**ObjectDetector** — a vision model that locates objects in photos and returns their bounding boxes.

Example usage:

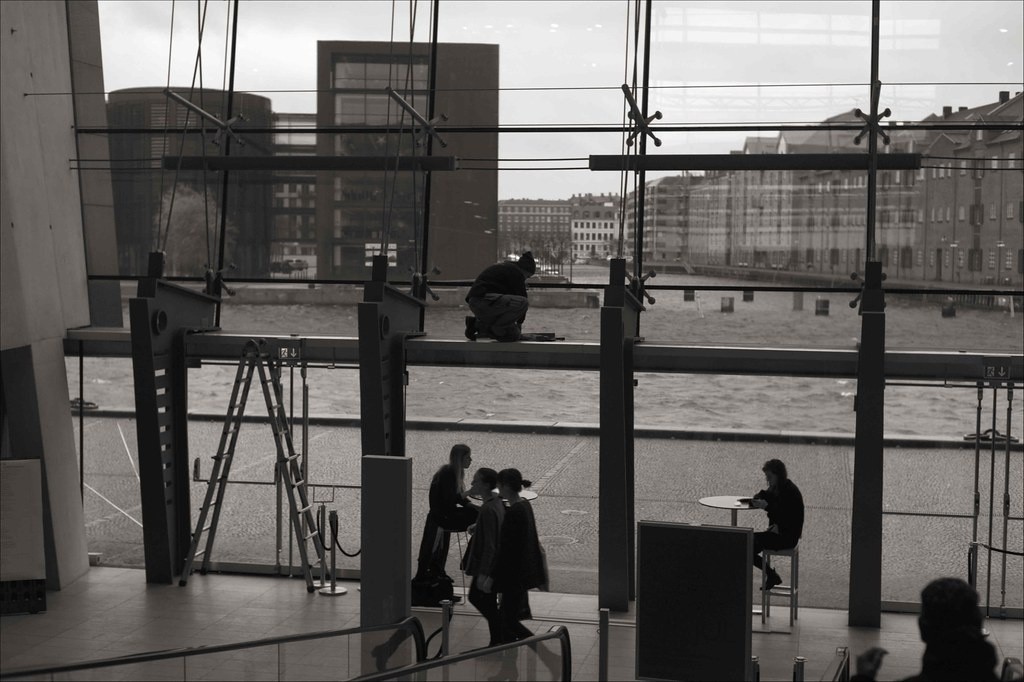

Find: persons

[464,249,538,344]
[737,459,805,591]
[459,467,512,662]
[848,576,1003,682]
[487,467,566,682]
[429,443,485,543]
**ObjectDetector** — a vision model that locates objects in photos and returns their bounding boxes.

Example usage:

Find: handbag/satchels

[536,541,550,592]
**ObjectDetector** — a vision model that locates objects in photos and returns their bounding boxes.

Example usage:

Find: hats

[514,251,536,274]
[763,458,787,478]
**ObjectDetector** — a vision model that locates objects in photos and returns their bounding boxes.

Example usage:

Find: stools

[438,528,469,606]
[761,544,799,624]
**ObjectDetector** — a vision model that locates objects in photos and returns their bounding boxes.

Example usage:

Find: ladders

[177,340,333,592]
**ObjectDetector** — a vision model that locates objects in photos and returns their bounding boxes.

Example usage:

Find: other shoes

[465,315,478,341]
[488,669,518,682]
[759,566,783,591]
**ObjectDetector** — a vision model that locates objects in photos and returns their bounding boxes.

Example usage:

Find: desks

[699,495,767,528]
[468,486,539,502]
